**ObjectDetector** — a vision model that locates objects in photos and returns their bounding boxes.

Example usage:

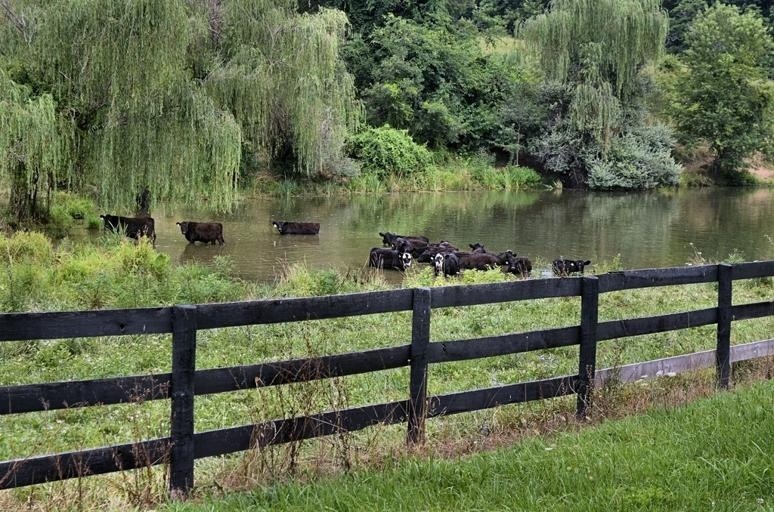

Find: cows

[550,258,591,277]
[176,221,225,246]
[271,220,321,236]
[368,231,532,277]
[100,213,156,249]
